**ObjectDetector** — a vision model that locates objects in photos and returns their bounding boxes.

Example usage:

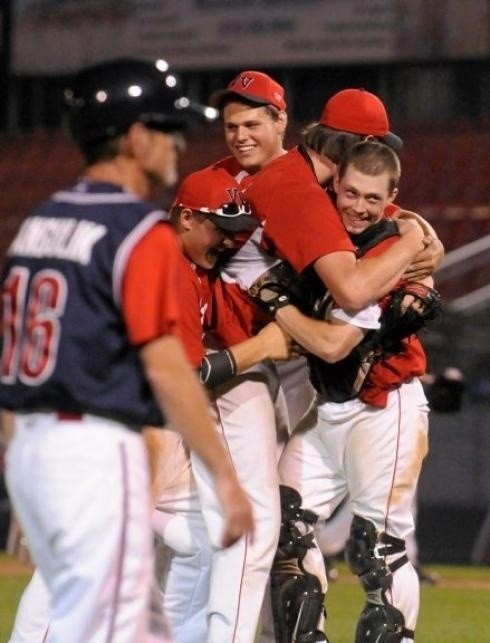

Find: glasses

[178,197,254,218]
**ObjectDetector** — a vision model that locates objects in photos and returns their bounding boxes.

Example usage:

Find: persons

[0,55,443,643]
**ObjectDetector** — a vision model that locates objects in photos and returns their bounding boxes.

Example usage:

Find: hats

[173,164,265,231]
[211,70,288,112]
[318,90,404,152]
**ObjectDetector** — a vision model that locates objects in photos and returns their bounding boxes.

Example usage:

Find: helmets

[65,57,221,135]
